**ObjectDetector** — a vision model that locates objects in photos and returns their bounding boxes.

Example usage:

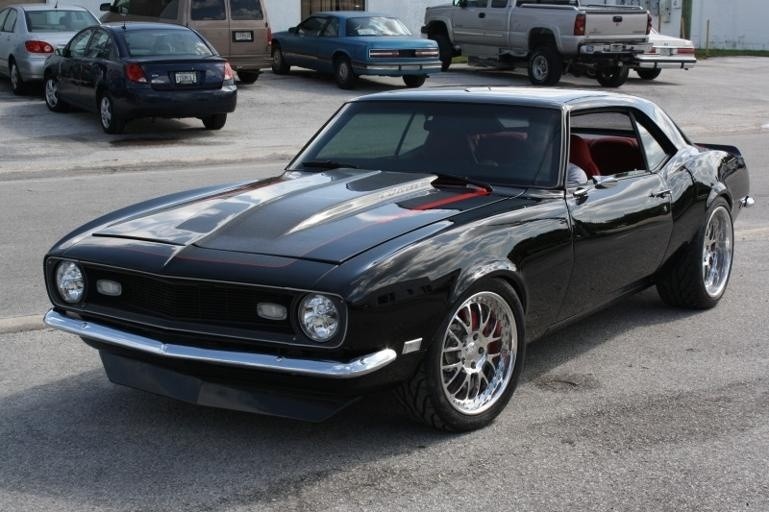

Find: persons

[493,114,588,188]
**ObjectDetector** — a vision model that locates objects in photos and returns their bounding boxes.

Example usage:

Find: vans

[97,0,273,84]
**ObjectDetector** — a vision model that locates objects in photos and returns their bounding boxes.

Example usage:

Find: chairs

[422,126,637,193]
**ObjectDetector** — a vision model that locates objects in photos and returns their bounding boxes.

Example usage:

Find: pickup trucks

[422,1,651,85]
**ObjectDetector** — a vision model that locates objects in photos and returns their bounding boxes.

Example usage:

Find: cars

[639,25,696,81]
[270,11,442,85]
[42,84,753,431]
[42,22,238,133]
[1,5,102,93]
